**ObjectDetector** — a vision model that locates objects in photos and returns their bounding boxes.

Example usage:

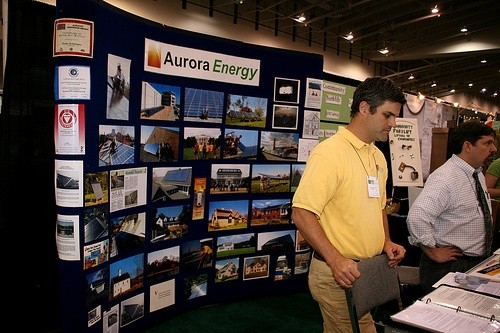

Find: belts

[313,251,360,263]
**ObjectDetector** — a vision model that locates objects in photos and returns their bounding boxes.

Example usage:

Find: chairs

[345,253,403,333]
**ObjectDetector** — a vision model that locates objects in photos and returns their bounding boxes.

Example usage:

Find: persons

[406,121,496,295]
[291,77,405,333]
[86,256,96,264]
[109,138,118,152]
[194,140,213,159]
[213,184,238,191]
[201,106,208,119]
[486,158,500,200]
[111,172,119,189]
[112,75,126,96]
[199,244,213,263]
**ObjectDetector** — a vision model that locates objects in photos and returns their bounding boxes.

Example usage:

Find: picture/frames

[295,230,311,254]
[272,104,299,130]
[242,255,270,280]
[273,77,301,105]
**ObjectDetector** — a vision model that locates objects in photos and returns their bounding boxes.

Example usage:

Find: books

[387,284,500,333]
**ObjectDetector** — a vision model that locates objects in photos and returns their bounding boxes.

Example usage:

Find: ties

[472,172,493,258]
